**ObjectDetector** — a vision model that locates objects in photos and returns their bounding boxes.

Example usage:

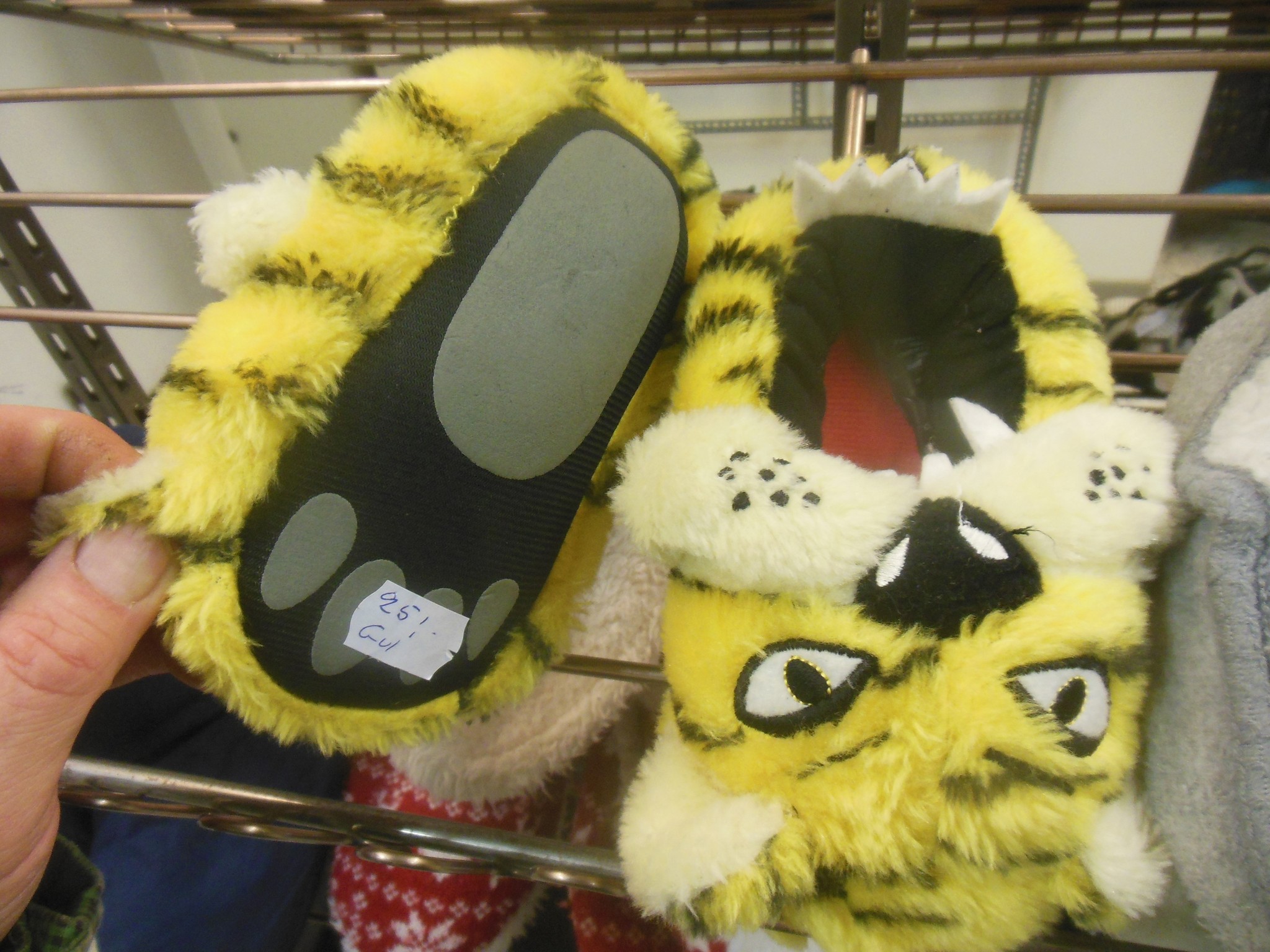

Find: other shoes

[578,727,728,952]
[31,51,724,758]
[612,136,1191,952]
[331,763,572,949]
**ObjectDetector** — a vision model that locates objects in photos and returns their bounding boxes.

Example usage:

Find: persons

[0,402,177,952]
